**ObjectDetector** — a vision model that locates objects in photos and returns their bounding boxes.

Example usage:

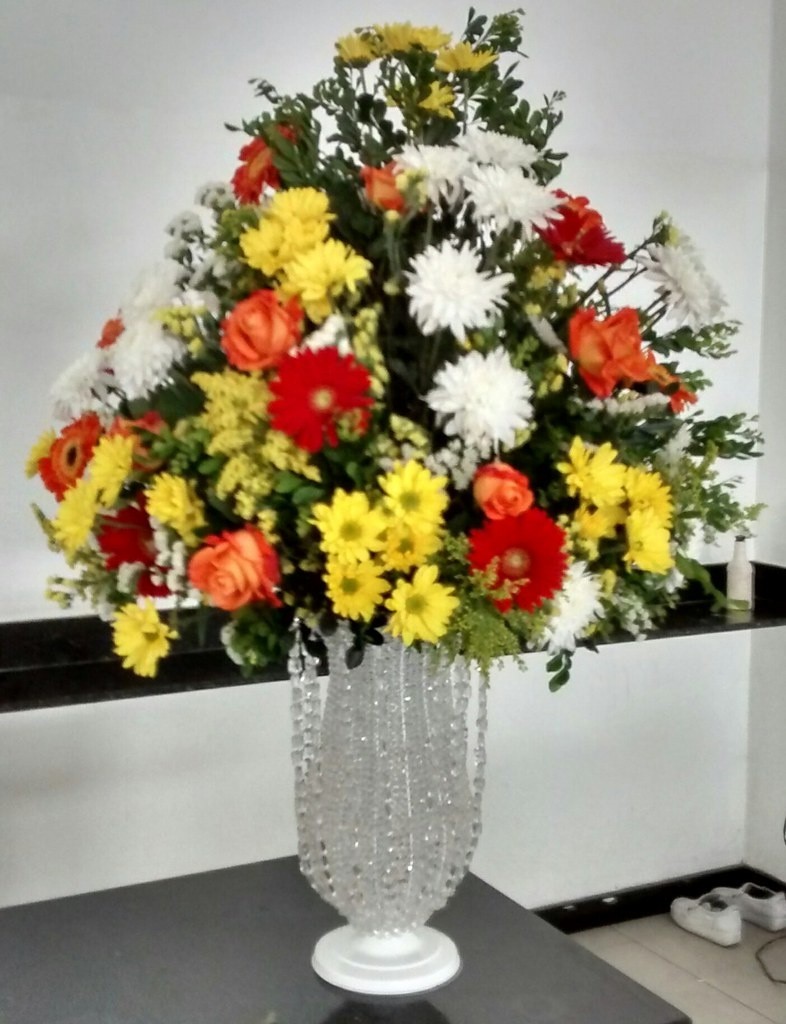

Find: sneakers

[713,881,786,932]
[672,892,741,948]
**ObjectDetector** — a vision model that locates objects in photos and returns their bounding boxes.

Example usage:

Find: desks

[0,850,697,1024]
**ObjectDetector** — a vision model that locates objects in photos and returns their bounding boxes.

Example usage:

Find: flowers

[18,4,771,692]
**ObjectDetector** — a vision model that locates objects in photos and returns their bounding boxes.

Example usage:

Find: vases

[285,600,491,1001]
